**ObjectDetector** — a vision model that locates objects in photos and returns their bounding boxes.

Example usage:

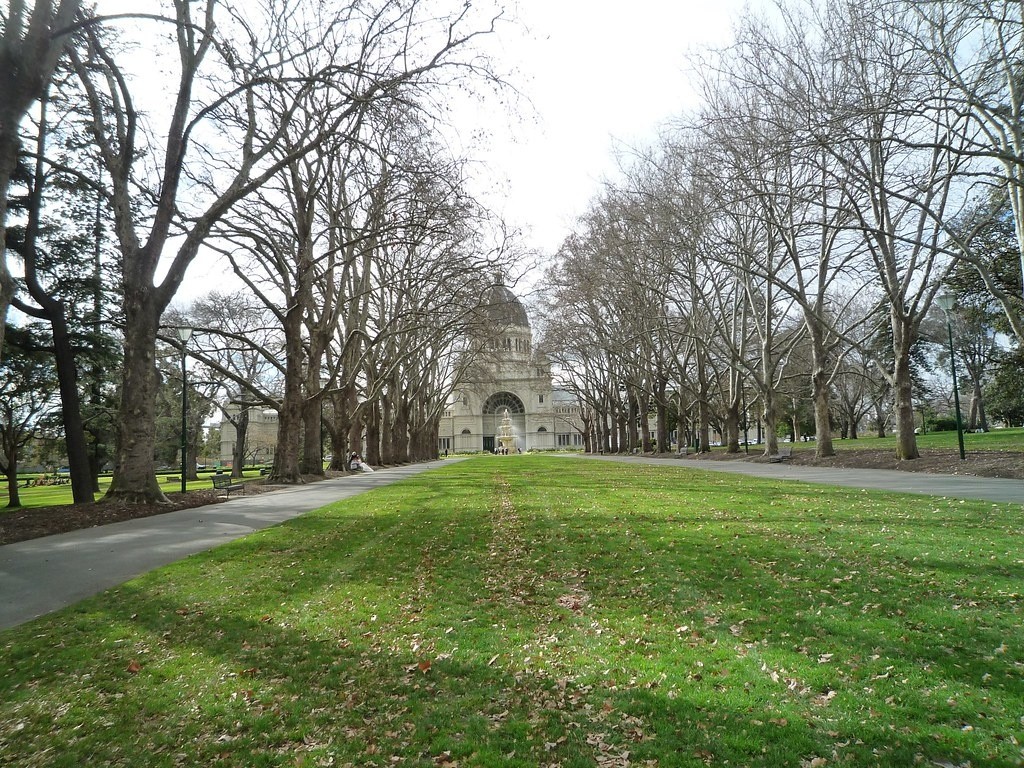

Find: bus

[59,466,70,472]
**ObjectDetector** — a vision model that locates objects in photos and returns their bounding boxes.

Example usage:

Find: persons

[445,449,448,457]
[652,439,656,449]
[518,447,522,452]
[494,447,508,455]
[639,441,642,450]
[350,451,361,470]
[964,426,983,434]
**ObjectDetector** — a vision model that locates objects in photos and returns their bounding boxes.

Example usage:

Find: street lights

[180,327,195,493]
[935,292,966,459]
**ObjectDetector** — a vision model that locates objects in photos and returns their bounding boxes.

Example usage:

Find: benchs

[260,469,270,476]
[674,447,688,458]
[349,460,364,472]
[210,474,245,499]
[167,477,181,482]
[769,447,793,463]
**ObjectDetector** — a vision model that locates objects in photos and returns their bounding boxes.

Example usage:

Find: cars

[738,439,758,445]
[324,455,332,462]
[708,442,722,447]
[196,463,205,469]
